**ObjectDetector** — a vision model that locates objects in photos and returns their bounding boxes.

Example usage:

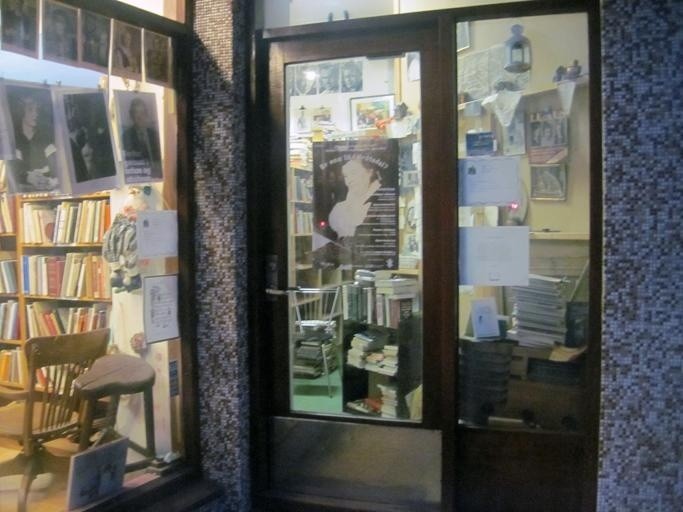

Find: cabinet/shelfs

[1,190,113,404]
[292,167,349,346]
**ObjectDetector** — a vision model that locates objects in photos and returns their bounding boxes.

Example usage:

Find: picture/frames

[349,94,395,131]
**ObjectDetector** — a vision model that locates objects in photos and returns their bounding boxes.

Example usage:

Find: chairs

[292,288,345,399]
[1,328,115,511]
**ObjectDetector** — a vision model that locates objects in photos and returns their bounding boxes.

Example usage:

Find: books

[294,174,421,417]
[1,194,112,394]
[503,271,571,348]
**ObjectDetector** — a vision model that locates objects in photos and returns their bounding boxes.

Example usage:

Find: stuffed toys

[100,187,160,293]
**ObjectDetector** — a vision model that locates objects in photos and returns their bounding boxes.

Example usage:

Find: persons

[10,91,60,190]
[122,99,162,176]
[342,62,362,92]
[1,0,37,48]
[46,9,75,60]
[289,67,316,96]
[80,15,106,66]
[320,64,338,94]
[113,31,140,74]
